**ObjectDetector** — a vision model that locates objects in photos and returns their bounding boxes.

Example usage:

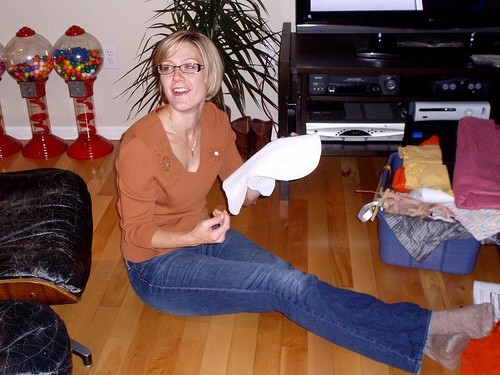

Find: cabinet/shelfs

[279,22,500,157]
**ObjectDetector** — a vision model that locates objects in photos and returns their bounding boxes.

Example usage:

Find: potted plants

[112,0,288,136]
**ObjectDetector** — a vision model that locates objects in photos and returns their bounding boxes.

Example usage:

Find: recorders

[426,69,500,101]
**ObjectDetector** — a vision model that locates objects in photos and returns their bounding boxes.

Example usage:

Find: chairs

[0,167,92,305]
[0,298,93,375]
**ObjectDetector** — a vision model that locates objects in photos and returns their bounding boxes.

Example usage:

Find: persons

[114,30,495,375]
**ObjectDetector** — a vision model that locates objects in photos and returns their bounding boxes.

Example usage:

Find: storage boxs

[376,153,480,275]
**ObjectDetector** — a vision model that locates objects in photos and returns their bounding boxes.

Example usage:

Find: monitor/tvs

[296,0,436,28]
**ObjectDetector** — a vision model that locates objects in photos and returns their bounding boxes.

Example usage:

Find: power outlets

[102,44,118,70]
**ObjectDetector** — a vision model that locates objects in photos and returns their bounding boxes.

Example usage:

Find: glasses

[155,62,205,75]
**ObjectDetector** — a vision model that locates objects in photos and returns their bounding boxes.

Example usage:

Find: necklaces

[167,106,199,161]
